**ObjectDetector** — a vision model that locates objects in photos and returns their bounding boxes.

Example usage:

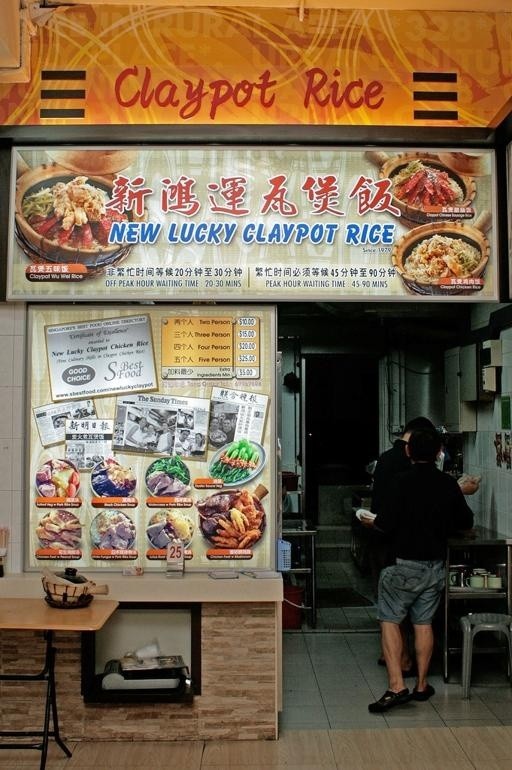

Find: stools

[460,613,512,701]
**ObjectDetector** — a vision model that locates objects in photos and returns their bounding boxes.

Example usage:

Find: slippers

[378,659,387,666]
[400,663,432,678]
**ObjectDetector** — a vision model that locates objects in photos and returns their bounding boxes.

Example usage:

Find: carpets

[316,587,375,608]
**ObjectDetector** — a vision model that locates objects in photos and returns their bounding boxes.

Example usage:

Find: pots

[42,567,109,603]
[198,484,269,548]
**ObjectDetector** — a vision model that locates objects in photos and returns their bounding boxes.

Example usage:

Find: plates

[207,440,266,488]
[90,509,137,550]
[37,509,83,550]
[36,458,81,498]
[146,509,196,551]
[90,460,138,498]
[44,595,94,609]
[144,456,193,498]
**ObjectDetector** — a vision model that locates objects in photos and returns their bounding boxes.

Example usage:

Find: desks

[443,526,511,683]
[0,598,119,770]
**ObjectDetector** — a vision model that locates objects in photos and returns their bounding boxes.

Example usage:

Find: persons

[361,426,475,712]
[367,416,479,678]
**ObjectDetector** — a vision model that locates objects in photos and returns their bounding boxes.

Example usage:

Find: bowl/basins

[391,221,490,292]
[14,163,150,265]
[379,151,476,225]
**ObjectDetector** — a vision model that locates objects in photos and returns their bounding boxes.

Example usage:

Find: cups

[448,564,502,589]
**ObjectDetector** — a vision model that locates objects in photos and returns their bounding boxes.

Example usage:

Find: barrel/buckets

[282,585,312,629]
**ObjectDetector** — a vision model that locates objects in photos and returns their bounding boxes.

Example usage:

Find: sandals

[413,683,435,702]
[367,687,412,712]
